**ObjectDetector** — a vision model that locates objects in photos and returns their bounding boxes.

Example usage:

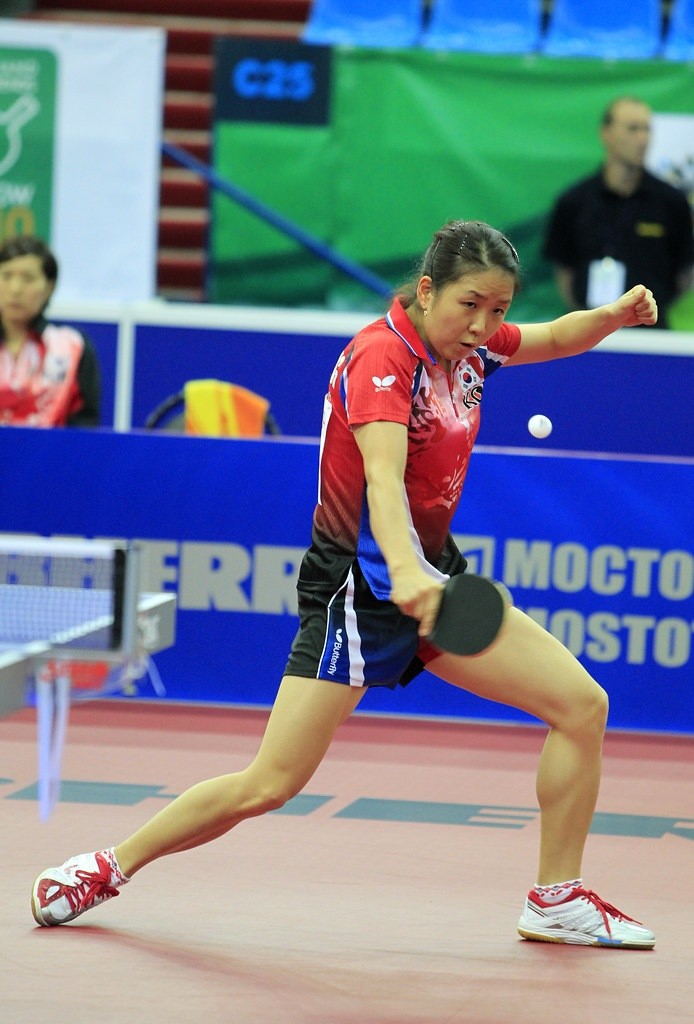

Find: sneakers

[517,889,656,951]
[31,849,120,927]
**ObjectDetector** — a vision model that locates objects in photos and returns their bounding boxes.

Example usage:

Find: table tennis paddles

[424,573,512,658]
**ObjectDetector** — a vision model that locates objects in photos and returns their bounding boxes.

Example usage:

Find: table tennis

[527,414,553,439]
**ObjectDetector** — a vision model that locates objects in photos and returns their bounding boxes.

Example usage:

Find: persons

[541,98,694,330]
[0,236,101,430]
[30,219,657,947]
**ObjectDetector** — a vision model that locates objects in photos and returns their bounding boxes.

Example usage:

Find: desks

[0,424,694,738]
[0,582,177,716]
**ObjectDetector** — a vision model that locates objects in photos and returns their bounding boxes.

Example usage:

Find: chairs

[144,379,279,438]
